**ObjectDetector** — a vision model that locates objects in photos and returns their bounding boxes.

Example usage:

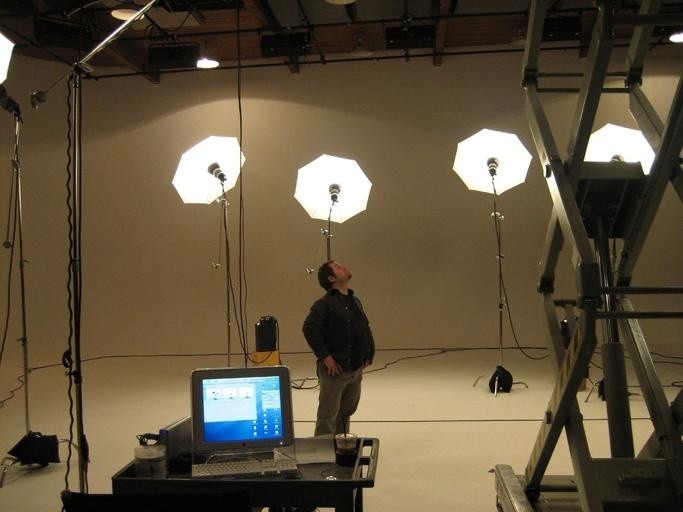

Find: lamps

[318,182,343,266]
[475,155,529,397]
[588,155,643,405]
[208,160,231,368]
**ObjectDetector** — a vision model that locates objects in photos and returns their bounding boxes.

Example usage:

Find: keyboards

[192,459,298,480]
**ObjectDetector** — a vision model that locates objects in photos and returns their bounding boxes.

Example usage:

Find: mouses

[285,470,302,481]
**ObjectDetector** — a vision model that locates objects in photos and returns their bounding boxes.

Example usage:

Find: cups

[334,433,357,453]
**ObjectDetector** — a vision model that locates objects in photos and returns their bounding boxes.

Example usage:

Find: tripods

[585,238,644,404]
[473,215,529,397]
[0,160,91,489]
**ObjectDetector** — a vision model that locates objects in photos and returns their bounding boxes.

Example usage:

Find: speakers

[255,323,277,352]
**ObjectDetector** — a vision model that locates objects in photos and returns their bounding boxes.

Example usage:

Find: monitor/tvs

[190,364,294,461]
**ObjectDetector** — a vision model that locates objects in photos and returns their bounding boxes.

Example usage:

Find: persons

[302,260,376,437]
